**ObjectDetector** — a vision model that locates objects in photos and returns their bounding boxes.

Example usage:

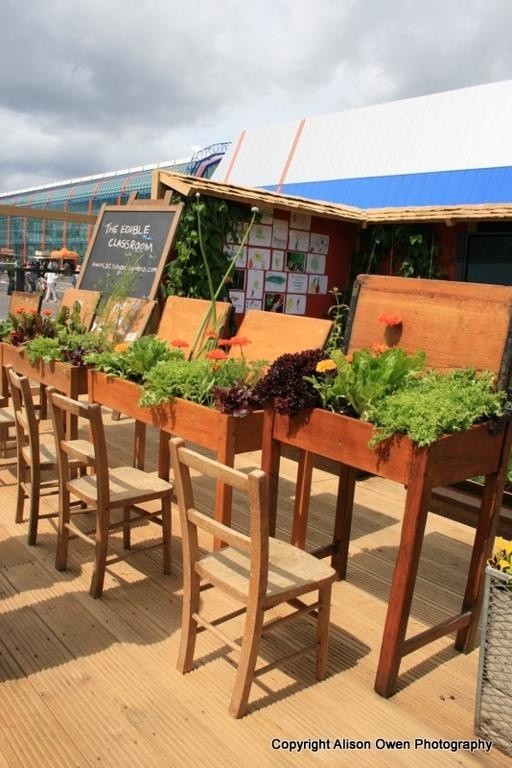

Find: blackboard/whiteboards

[74,203,182,301]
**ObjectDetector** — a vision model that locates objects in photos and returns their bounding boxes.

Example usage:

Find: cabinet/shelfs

[262,274,512,699]
[89,296,333,551]
[0,289,156,479]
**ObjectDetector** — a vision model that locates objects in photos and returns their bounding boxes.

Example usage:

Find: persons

[6,260,81,303]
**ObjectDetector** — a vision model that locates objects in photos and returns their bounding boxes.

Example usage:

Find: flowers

[8,306,54,345]
[309,284,429,422]
[139,193,269,406]
[486,536,512,579]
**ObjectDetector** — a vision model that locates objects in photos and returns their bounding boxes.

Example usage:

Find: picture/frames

[222,205,330,317]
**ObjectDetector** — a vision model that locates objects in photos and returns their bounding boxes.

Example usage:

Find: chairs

[45,386,174,598]
[169,436,338,718]
[0,406,40,468]
[5,364,93,545]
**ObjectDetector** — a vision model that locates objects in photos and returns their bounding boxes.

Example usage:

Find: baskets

[474,567,512,759]
